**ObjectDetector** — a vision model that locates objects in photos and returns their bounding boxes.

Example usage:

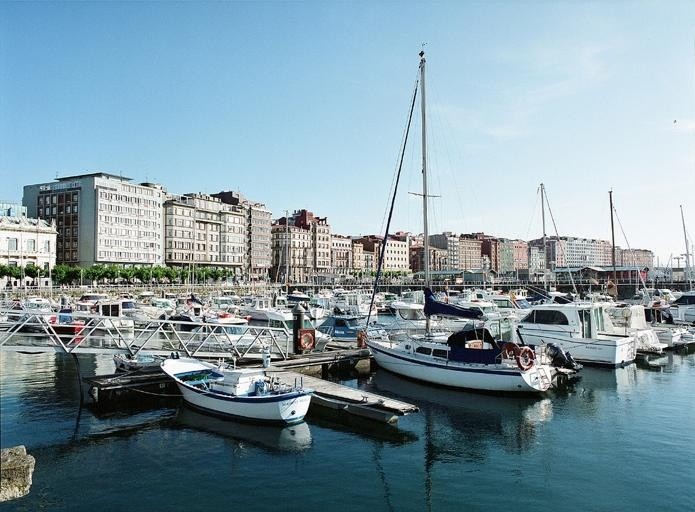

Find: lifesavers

[516,346,534,370]
[502,342,520,359]
[300,333,314,350]
[222,314,231,318]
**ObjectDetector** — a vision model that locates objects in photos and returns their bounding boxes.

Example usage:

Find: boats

[168,400,314,458]
